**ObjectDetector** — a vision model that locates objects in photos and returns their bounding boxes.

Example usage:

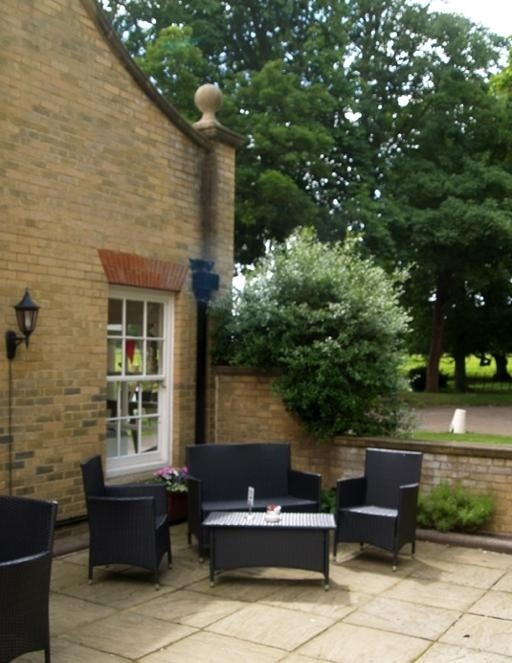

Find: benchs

[185,441,322,564]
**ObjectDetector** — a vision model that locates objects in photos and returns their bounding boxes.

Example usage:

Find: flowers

[153,466,188,493]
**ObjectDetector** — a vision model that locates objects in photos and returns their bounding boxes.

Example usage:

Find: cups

[266,510,281,515]
[264,515,280,522]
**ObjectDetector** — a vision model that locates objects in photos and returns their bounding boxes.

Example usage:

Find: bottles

[134,383,140,399]
[129,392,139,424]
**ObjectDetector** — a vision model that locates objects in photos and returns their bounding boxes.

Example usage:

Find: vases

[168,493,187,526]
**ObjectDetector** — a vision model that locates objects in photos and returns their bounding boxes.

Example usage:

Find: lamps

[5,287,41,360]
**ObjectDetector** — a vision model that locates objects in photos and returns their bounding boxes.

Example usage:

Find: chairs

[333,447,423,572]
[79,453,172,590]
[0,494,59,663]
